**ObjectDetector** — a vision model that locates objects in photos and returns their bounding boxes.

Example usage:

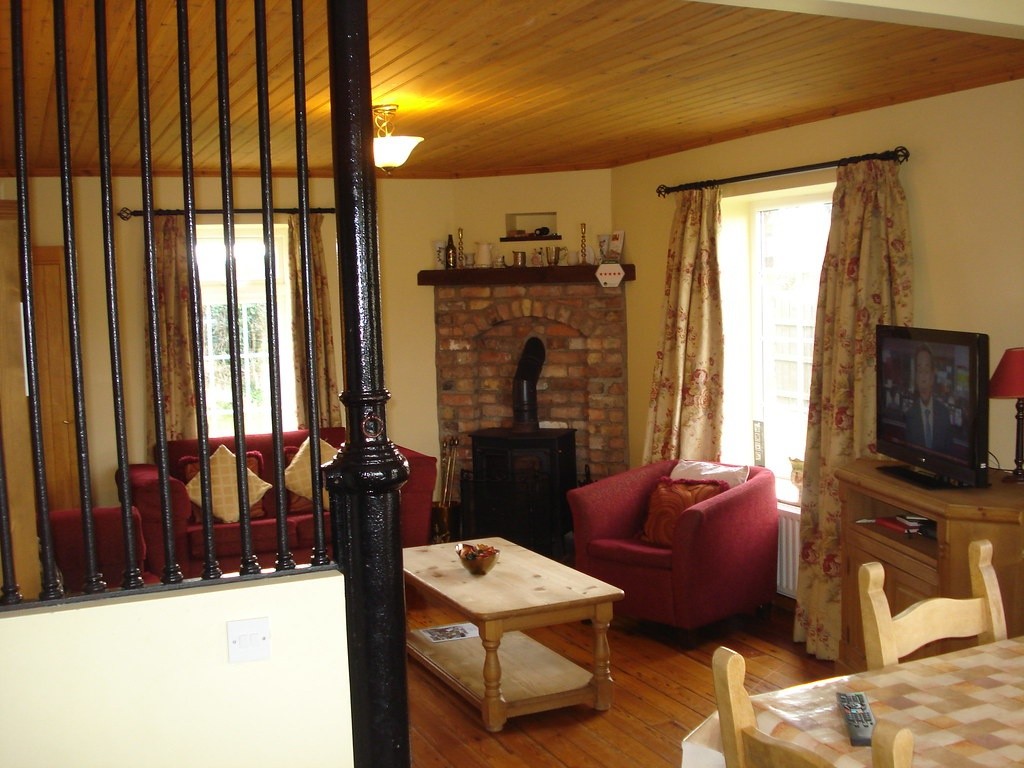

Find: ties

[924,408,932,450]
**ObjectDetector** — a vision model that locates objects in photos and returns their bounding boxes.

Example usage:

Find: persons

[904,345,953,453]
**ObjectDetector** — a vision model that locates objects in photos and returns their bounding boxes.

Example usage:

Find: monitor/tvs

[874,325,993,490]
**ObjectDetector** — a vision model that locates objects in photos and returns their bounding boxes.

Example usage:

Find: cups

[545,246,568,266]
[513,251,526,268]
[432,239,446,270]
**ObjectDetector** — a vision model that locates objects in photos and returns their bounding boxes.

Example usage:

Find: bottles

[444,234,457,271]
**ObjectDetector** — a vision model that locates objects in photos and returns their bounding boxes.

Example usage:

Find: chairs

[858,539,1009,669]
[711,646,915,768]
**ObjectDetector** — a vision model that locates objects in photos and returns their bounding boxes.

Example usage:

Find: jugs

[473,241,495,268]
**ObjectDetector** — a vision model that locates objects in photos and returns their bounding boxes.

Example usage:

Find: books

[876,512,928,534]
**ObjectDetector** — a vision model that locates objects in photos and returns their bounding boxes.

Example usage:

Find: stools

[48,504,148,595]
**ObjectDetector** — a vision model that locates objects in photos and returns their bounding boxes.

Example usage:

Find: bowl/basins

[455,543,501,576]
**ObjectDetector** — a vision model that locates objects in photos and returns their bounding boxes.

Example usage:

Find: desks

[832,458,1024,675]
[403,535,624,733]
[682,634,1024,768]
[467,427,579,554]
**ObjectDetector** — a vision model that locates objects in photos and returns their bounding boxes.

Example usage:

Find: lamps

[989,348,1024,483]
[372,103,426,177]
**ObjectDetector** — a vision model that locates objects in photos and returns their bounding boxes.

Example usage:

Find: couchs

[566,458,779,650]
[115,425,437,579]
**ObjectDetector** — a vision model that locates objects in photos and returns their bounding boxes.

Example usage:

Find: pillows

[670,459,750,489]
[282,446,322,512]
[179,450,268,524]
[185,444,272,523]
[284,436,339,512]
[639,474,730,548]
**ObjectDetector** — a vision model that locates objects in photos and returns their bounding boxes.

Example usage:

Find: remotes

[836,691,876,746]
